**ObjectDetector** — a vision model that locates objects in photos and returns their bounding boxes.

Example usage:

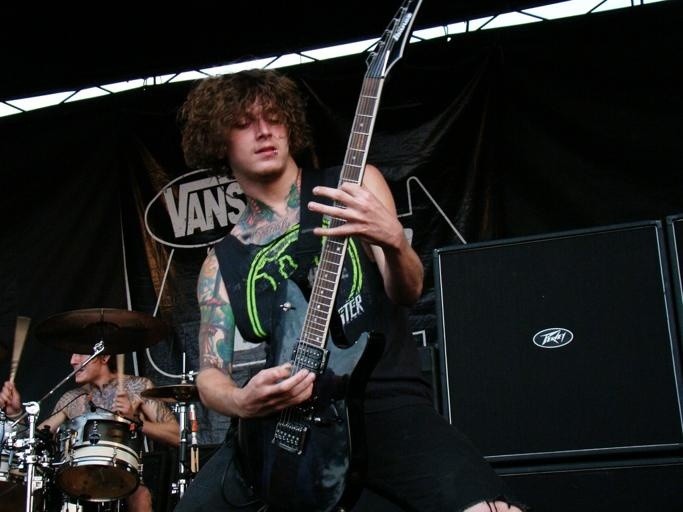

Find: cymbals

[138,381,198,406]
[32,307,158,352]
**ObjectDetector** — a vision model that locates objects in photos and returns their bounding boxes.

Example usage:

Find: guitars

[227,0,422,512]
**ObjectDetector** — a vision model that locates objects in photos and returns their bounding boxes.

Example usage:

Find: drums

[55,411,145,511]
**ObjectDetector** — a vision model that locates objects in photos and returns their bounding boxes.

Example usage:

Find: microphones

[93,325,138,359]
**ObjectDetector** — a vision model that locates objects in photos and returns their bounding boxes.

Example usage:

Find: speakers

[431,220,681,463]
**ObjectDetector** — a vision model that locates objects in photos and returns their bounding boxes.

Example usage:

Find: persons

[174,69,423,512]
[0,340,180,512]
